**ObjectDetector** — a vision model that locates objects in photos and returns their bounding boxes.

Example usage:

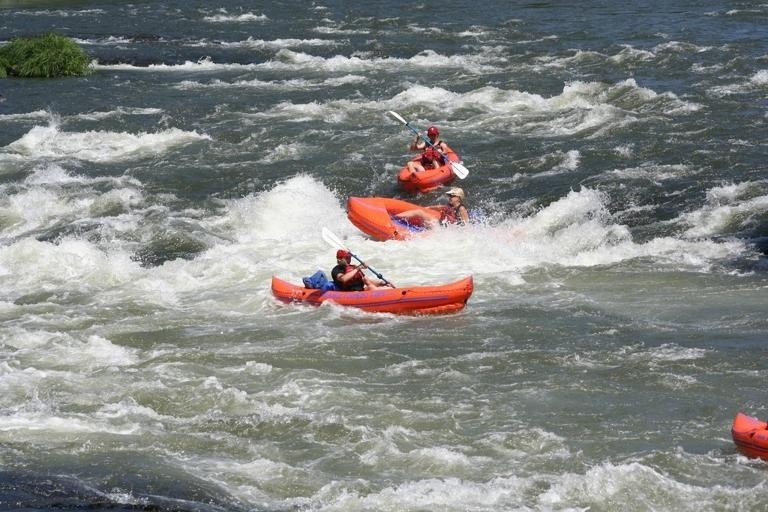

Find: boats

[269,274,476,316]
[397,142,465,193]
[730,410,768,461]
[345,189,450,242]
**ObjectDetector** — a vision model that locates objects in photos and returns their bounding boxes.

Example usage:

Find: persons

[331,249,395,291]
[441,187,469,224]
[407,125,448,173]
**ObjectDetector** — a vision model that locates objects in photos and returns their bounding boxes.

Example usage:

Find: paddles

[322,227,396,288]
[389,110,469,180]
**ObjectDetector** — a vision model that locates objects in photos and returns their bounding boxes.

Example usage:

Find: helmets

[337,249,351,258]
[426,126,440,136]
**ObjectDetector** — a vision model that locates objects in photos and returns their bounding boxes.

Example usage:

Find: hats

[445,186,464,201]
